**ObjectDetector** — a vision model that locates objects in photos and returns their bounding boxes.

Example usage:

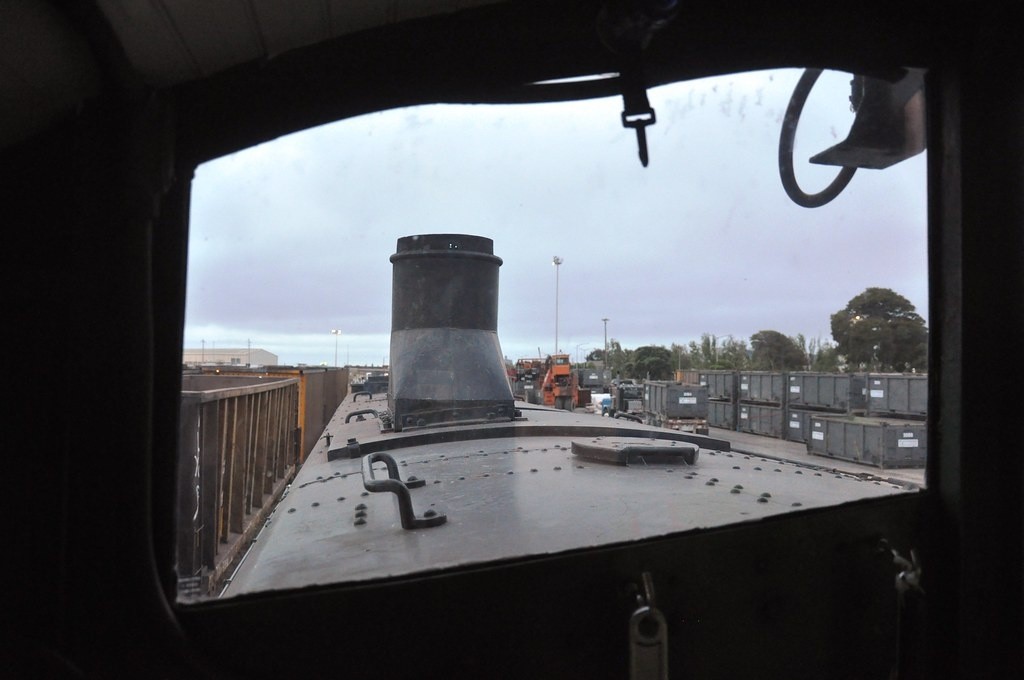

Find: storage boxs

[786,373,865,414]
[785,409,826,443]
[645,415,709,436]
[808,413,925,470]
[697,369,737,403]
[865,373,928,417]
[737,370,787,408]
[707,401,737,431]
[641,379,708,420]
[737,403,787,440]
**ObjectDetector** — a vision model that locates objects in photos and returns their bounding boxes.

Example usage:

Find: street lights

[553,256,564,363]
[602,318,611,368]
[331,329,342,365]
